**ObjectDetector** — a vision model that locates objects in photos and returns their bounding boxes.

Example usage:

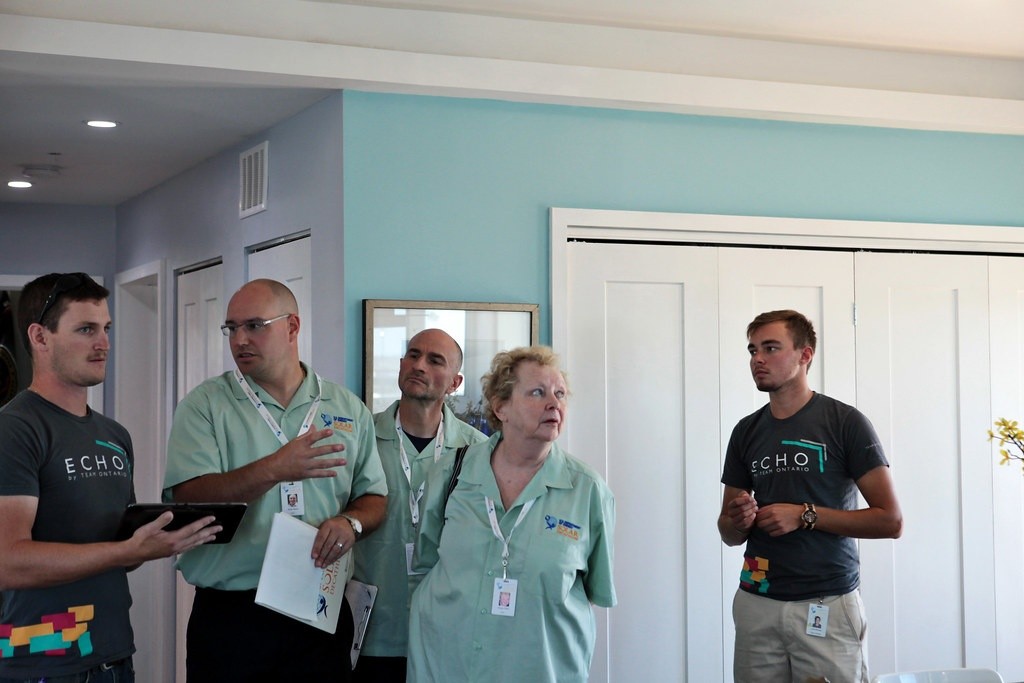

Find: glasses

[38,273,87,323]
[220,314,290,336]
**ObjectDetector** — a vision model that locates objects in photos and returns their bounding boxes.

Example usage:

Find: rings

[335,543,344,550]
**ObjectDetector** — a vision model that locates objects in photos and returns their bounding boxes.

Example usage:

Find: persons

[812,616,821,628]
[0,272,223,683]
[717,309,903,683]
[287,495,298,508]
[160,279,388,683]
[499,592,511,607]
[351,328,490,683]
[405,346,620,683]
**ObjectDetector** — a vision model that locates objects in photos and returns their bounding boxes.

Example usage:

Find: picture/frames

[361,298,540,416]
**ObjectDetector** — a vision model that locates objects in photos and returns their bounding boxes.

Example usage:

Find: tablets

[115,503,249,544]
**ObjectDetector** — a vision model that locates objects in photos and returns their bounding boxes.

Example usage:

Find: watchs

[337,513,363,542]
[801,503,817,530]
